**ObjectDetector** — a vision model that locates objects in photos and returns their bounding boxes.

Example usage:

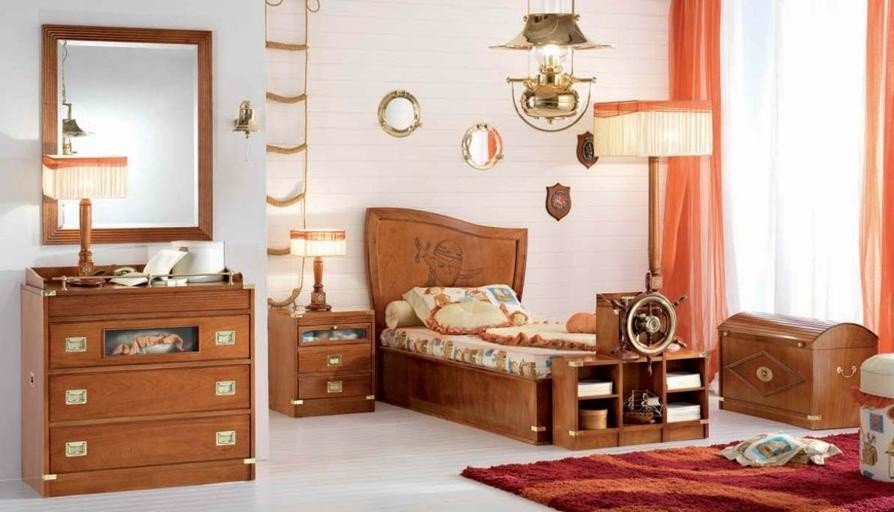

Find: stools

[856,351,893,483]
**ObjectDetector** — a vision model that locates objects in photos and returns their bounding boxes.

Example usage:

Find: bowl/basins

[170,239,225,282]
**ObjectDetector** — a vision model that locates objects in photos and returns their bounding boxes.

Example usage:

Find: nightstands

[268,310,378,417]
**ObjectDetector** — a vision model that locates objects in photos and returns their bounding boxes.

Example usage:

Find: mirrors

[38,21,214,246]
[377,90,423,138]
[461,122,504,171]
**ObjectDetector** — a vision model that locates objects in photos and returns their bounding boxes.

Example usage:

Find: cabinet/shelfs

[22,269,260,499]
[555,350,714,448]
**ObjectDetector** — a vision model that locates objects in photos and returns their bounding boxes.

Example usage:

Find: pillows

[426,301,512,336]
[403,279,530,337]
[381,298,422,330]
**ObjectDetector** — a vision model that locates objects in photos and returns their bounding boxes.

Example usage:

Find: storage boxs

[716,311,882,433]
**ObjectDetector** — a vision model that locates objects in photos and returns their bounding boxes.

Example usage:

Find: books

[666,371,701,390]
[667,402,701,423]
[578,379,613,397]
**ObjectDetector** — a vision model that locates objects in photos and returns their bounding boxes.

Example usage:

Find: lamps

[42,155,134,286]
[230,100,260,175]
[593,98,717,301]
[488,3,613,134]
[292,226,345,312]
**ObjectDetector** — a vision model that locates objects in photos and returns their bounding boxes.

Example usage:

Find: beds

[364,207,710,448]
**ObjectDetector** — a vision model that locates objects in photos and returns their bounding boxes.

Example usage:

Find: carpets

[452,431,894,512]
